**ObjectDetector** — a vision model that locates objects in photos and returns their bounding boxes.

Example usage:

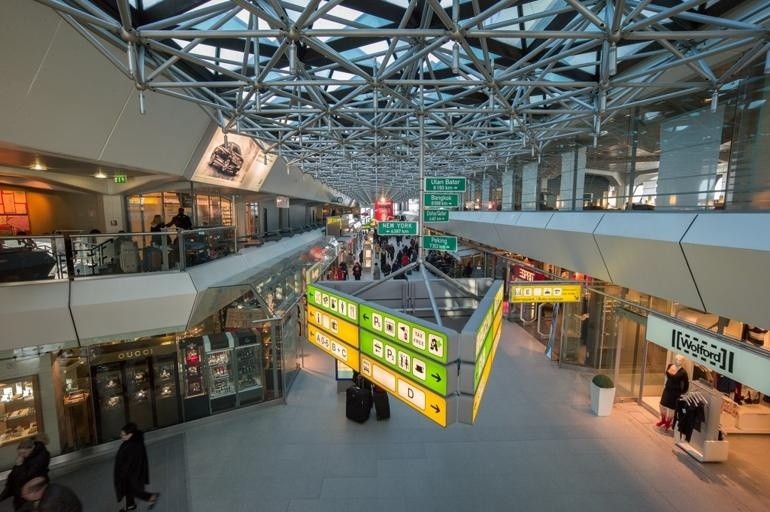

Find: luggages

[346,385,390,423]
[119,240,163,272]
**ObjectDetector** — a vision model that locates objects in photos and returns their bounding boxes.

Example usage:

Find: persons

[114,422,159,512]
[359,250,363,263]
[353,263,362,280]
[425,250,473,279]
[21,477,82,512]
[166,208,191,230]
[374,229,418,279]
[340,262,348,280]
[0,440,50,512]
[656,354,689,430]
[151,215,165,245]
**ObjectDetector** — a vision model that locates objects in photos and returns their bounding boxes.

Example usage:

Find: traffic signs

[377,177,467,253]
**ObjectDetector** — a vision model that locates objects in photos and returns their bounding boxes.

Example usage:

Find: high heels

[656,417,671,431]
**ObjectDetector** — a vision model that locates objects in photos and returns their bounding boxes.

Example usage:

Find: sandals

[147,492,160,509]
[119,500,137,512]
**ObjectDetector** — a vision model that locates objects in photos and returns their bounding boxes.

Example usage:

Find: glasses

[120,434,126,437]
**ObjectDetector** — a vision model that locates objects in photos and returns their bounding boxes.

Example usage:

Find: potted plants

[591,374,616,417]
